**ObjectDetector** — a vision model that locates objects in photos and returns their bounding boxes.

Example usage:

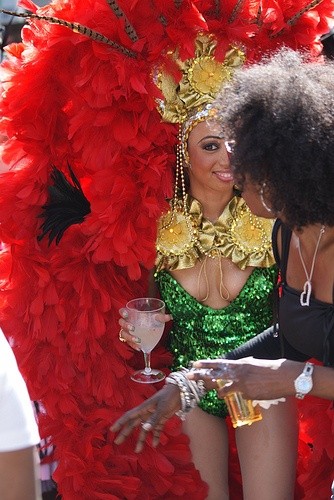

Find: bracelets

[162,368,209,412]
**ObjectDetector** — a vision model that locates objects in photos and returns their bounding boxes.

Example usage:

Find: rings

[117,327,132,341]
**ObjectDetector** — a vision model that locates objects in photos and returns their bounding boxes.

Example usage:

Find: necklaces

[293,227,324,307]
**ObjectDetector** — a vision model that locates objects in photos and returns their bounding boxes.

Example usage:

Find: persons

[119,85,298,500]
[110,48,333,452]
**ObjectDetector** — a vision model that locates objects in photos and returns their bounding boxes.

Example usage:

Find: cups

[216,356,262,428]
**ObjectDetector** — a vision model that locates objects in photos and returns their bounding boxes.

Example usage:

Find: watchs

[293,362,313,399]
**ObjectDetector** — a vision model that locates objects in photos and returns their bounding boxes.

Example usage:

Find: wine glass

[126,298,166,383]
